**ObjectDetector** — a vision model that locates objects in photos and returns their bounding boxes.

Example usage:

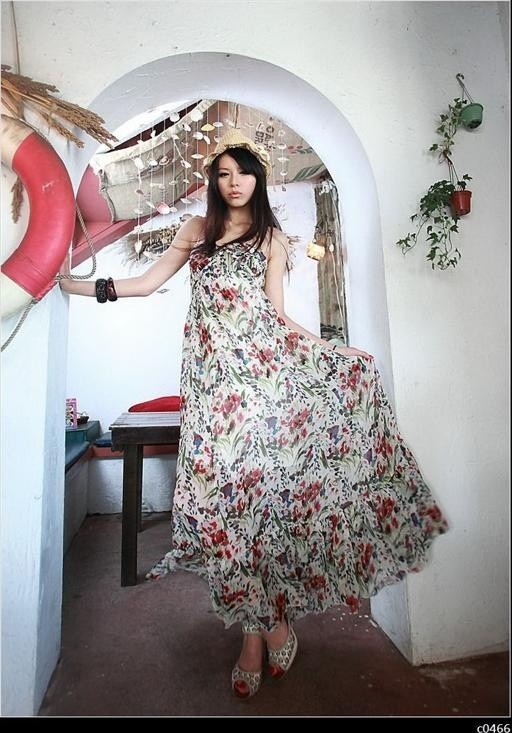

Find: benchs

[64,439,90,555]
[91,431,180,520]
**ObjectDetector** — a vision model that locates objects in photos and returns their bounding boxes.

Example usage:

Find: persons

[58,127,448,703]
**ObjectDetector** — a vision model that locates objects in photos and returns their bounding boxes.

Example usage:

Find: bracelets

[106,278,117,302]
[333,345,337,350]
[95,279,107,303]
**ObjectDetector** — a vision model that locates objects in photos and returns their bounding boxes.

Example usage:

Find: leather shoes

[268,620,301,681]
[230,659,268,699]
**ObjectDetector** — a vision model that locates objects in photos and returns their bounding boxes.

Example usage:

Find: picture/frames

[66,398,78,429]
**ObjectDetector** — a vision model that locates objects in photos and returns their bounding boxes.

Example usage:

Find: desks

[109,410,184,589]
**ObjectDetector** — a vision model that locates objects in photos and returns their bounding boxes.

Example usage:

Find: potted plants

[395,98,484,273]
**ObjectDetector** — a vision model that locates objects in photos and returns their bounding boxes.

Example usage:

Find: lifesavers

[0,114,75,324]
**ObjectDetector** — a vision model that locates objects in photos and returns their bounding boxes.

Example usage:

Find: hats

[202,127,273,178]
